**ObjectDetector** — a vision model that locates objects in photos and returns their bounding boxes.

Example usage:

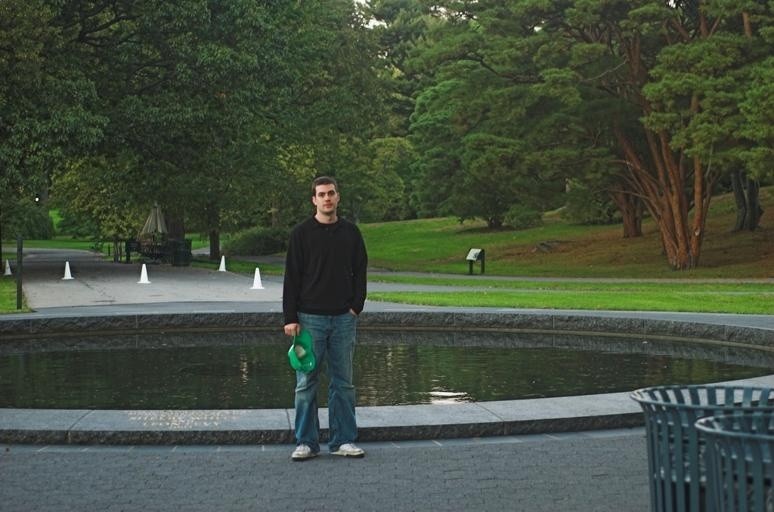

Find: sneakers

[328,443,365,458]
[292,443,321,460]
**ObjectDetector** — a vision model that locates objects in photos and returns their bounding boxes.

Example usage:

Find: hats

[287,326,316,375]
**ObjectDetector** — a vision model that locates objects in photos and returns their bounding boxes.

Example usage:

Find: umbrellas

[140,206,168,236]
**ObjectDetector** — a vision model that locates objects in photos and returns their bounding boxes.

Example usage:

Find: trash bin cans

[168,238,191,267]
[628,386,774,511]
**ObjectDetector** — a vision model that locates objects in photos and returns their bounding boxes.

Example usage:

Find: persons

[283,175,366,460]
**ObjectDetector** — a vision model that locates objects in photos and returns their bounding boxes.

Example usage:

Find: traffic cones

[137,263,152,284]
[218,255,227,272]
[4,259,13,276]
[250,267,264,290]
[61,261,74,280]
